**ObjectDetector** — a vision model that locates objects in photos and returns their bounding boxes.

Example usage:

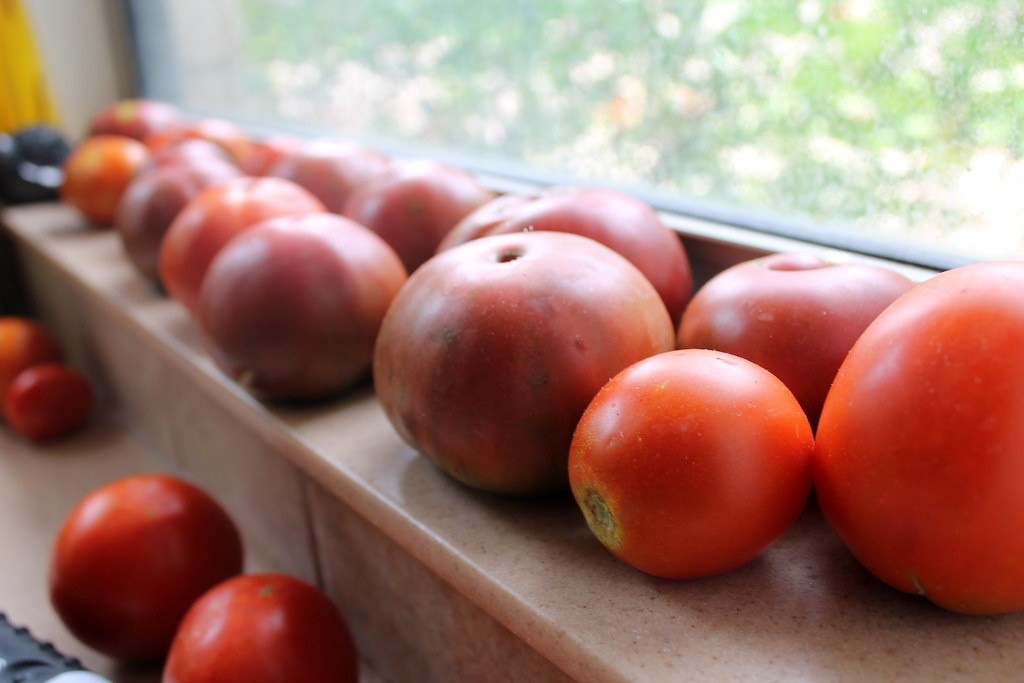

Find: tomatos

[65,103,1024,620]
[0,317,93,443]
[47,472,362,683]
